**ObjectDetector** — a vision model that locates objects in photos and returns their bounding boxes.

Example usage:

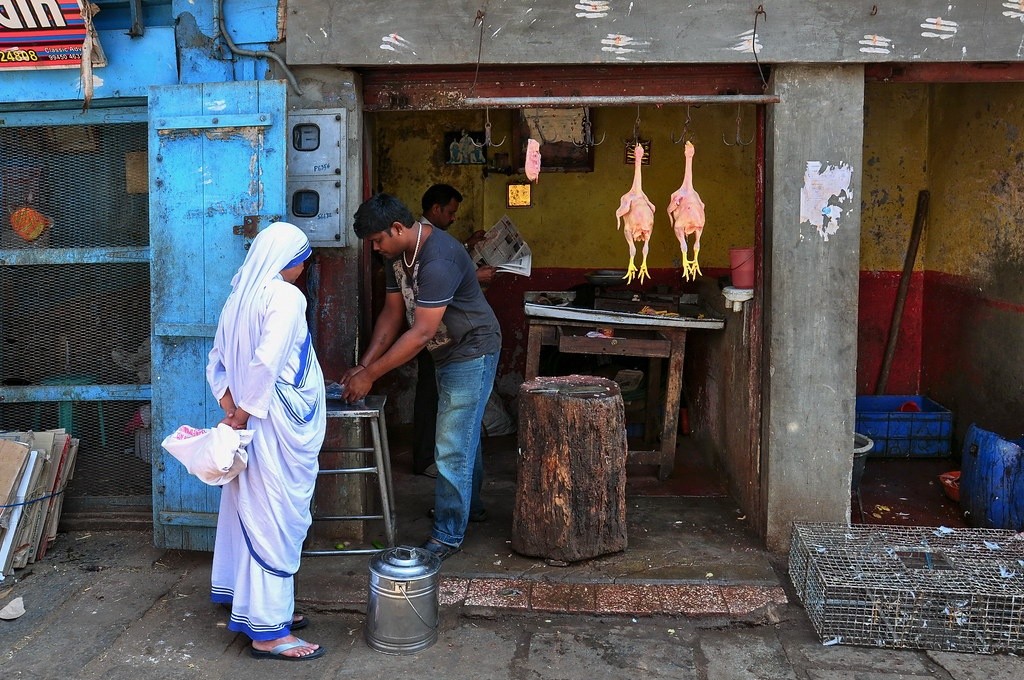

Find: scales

[584,270,640,313]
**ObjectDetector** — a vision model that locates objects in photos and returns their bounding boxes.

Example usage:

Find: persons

[206,222,327,661]
[339,194,502,560]
[412,184,501,478]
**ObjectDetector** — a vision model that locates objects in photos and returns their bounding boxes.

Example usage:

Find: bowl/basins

[896,401,920,412]
[940,471,960,502]
[523,290,577,302]
[584,270,628,286]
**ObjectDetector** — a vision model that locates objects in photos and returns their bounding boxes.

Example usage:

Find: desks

[301,394,398,557]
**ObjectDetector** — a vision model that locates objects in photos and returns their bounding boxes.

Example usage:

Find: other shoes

[424,463,439,478]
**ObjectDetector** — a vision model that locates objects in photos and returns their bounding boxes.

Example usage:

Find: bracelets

[357,363,366,368]
[463,241,470,251]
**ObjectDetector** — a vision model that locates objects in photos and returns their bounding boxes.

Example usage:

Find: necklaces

[403,222,422,268]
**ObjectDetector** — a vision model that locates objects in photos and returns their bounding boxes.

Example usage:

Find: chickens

[616,140,705,285]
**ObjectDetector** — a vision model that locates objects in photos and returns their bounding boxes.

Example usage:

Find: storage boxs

[856,394,953,462]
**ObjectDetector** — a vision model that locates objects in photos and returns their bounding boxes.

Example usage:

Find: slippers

[289,615,307,630]
[251,636,326,660]
[420,538,460,562]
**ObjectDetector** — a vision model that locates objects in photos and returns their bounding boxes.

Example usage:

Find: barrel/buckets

[366,544,442,656]
[850,432,874,490]
[729,247,754,289]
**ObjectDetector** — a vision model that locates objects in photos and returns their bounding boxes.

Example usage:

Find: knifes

[526,385,607,395]
